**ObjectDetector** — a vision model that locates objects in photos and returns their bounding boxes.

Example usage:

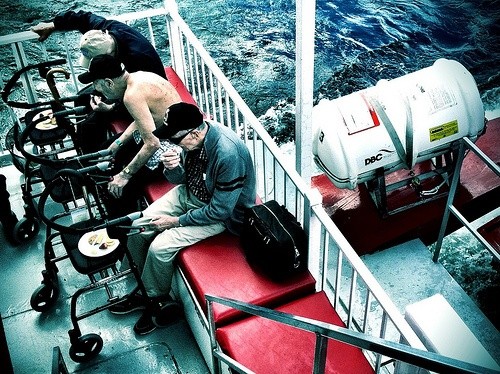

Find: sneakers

[109,293,175,336]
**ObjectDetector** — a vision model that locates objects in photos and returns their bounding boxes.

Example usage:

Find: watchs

[123,167,135,176]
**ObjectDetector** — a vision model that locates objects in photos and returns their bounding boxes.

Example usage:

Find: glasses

[167,127,197,146]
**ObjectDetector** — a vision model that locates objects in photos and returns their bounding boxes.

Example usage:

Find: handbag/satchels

[238,200,308,279]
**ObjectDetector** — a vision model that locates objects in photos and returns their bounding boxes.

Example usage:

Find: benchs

[102,68,375,374]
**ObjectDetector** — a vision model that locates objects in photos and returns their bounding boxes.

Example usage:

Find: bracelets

[115,139,123,147]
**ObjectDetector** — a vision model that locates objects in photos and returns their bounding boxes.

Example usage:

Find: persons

[78,53,184,246]
[107,102,256,335]
[26,11,168,154]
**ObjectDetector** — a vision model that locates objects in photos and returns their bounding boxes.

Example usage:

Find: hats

[78,54,126,84]
[152,102,204,139]
[79,29,115,68]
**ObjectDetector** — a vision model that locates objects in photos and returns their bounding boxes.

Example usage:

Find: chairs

[1,60,179,367]
